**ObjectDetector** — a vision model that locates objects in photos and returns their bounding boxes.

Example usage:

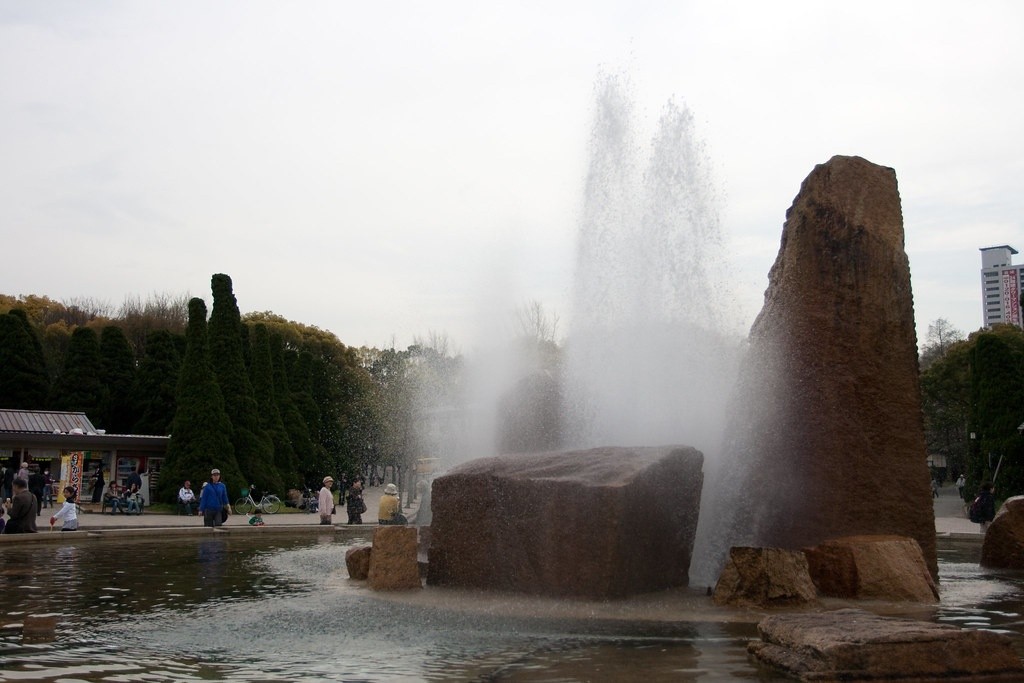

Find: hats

[385,483,397,495]
[211,469,220,475]
[323,476,333,483]
[202,482,208,488]
[185,481,191,486]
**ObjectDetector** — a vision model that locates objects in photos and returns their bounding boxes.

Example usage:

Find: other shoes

[43,506,47,508]
[51,504,53,508]
[137,513,141,516]
[110,512,116,515]
[188,513,193,516]
[37,512,40,516]
[127,512,131,516]
[120,511,125,515]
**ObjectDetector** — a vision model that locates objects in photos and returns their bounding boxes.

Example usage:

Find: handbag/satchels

[331,506,336,514]
[3,518,26,534]
[221,508,228,523]
[360,499,367,513]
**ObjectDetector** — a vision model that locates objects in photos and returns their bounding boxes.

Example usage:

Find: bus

[413,458,441,474]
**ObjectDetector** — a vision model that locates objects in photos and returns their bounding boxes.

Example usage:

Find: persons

[967,483,996,533]
[89,464,105,505]
[352,474,367,483]
[248,509,264,526]
[931,478,938,499]
[409,479,432,524]
[318,475,336,525]
[338,471,347,505]
[345,478,367,524]
[198,469,232,526]
[178,481,196,516]
[50,485,78,531]
[0,476,38,534]
[0,462,54,516]
[378,483,408,524]
[297,488,318,514]
[107,480,126,515]
[124,466,143,514]
[955,473,966,499]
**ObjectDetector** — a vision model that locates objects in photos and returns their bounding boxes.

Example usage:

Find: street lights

[969,431,976,455]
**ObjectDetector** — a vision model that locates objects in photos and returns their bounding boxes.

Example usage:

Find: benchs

[102,492,144,512]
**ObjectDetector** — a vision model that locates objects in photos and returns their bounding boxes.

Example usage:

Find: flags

[56,451,85,503]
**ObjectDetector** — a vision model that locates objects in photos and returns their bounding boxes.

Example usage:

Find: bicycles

[234,484,281,515]
